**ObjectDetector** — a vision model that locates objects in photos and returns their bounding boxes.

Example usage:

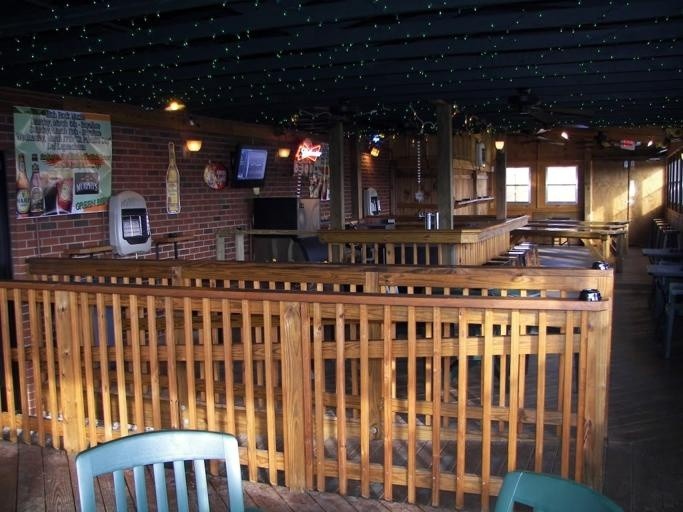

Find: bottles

[166,143,181,215]
[16,151,44,218]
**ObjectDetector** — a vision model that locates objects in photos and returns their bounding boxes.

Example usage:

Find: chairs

[493,469,624,510]
[73,428,244,511]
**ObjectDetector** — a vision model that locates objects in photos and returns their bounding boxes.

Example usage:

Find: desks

[510,216,630,271]
[640,247,683,360]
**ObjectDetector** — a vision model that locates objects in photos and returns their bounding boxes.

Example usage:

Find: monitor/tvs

[371,201,377,211]
[123,217,142,238]
[230,143,269,188]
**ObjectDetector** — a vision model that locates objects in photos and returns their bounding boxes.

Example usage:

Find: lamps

[369,147,380,158]
[273,145,291,160]
[180,140,202,160]
[490,141,504,167]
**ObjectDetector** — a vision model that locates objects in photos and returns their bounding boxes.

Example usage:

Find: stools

[483,241,538,268]
[651,217,679,247]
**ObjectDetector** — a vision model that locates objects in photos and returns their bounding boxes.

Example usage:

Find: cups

[42,170,74,215]
